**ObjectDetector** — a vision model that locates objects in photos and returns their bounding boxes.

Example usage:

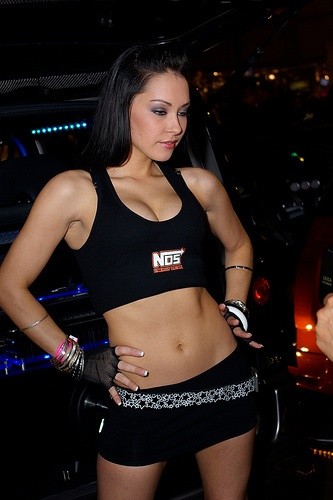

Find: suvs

[0,104,226,387]
